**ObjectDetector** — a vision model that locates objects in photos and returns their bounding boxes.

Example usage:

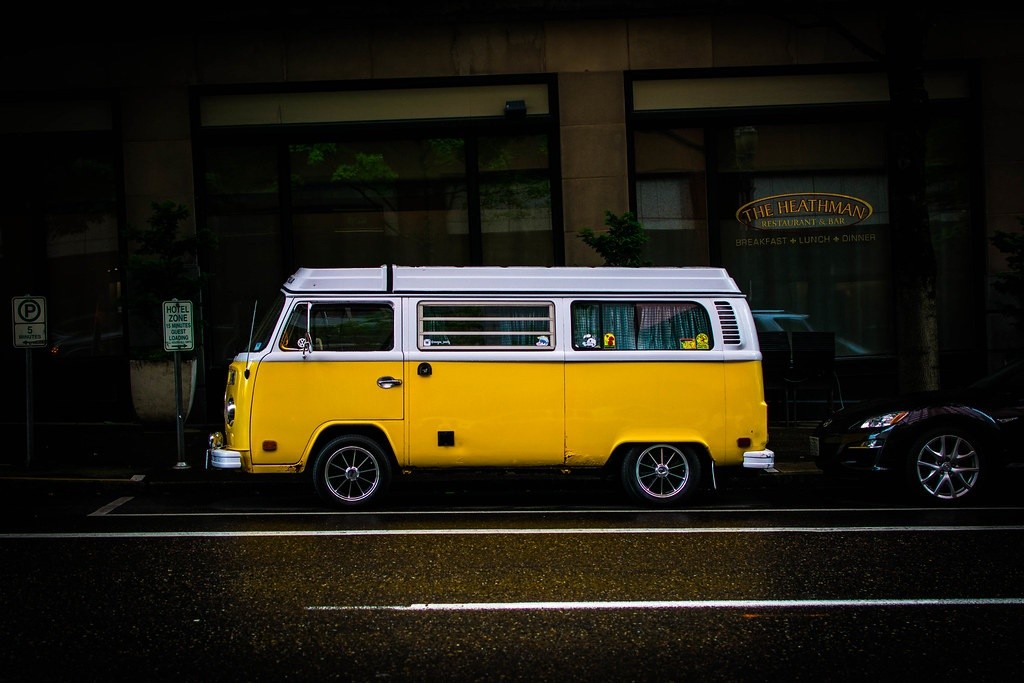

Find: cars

[35,309,128,358]
[750,307,872,356]
[808,358,1024,506]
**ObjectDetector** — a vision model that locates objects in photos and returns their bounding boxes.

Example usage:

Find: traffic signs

[163,300,194,352]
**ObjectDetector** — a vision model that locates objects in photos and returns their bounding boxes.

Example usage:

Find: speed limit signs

[13,324,47,347]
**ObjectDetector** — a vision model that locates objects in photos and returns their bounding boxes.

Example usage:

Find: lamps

[735,127,759,168]
[505,100,528,114]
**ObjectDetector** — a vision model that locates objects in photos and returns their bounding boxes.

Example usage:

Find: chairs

[758,331,791,426]
[790,332,843,425]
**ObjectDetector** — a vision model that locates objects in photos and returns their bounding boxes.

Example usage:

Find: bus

[202,258,777,509]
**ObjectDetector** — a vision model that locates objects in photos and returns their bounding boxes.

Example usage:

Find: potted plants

[122,201,222,428]
